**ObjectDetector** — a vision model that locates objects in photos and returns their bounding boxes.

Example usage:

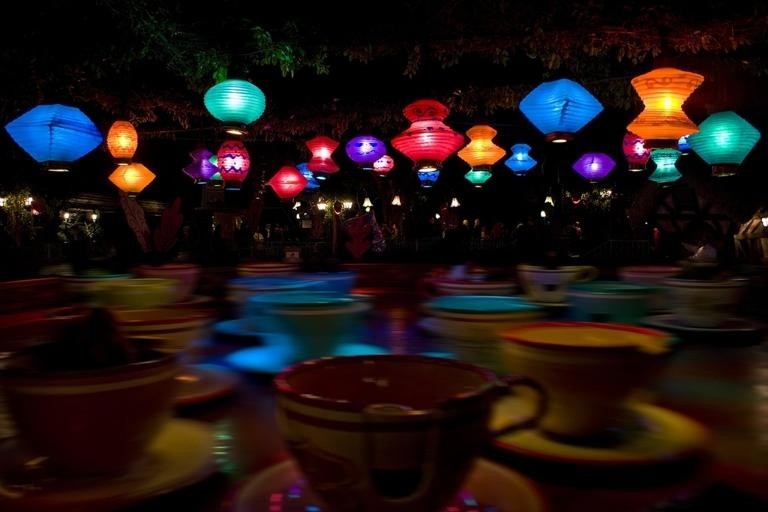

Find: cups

[1,339,182,483]
[225,262,391,305]
[1,309,91,342]
[421,275,541,362]
[106,309,215,382]
[250,289,363,359]
[516,263,747,327]
[1,261,200,311]
[494,322,678,443]
[645,310,755,336]
[269,352,548,510]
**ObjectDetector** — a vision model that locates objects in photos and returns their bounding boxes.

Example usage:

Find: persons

[18,307,131,368]
[69,225,85,241]
[674,239,726,265]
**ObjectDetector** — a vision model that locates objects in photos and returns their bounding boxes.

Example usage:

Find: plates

[483,374,714,468]
[164,363,243,406]
[224,342,382,377]
[228,452,539,511]
[1,413,230,511]
[135,297,212,309]
[207,315,260,337]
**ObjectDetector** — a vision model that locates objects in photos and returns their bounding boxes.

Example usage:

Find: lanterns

[5,65,763,199]
